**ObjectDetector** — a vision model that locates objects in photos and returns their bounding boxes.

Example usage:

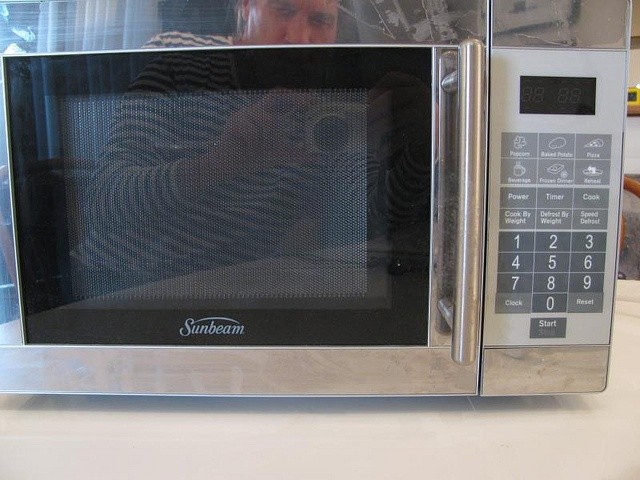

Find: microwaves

[0,2,634,399]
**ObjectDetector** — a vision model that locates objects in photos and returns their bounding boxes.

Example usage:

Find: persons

[70,1,433,299]
